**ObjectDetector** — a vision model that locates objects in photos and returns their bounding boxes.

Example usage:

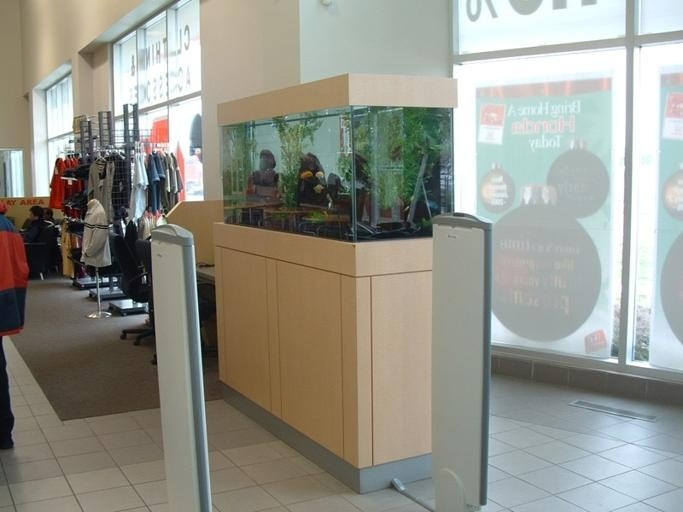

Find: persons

[24,205,55,243]
[0,198,29,449]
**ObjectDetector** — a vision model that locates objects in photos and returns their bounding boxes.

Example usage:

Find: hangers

[51,140,180,169]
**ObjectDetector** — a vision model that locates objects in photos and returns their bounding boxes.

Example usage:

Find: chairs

[134,239,216,366]
[109,232,155,345]
[24,242,63,280]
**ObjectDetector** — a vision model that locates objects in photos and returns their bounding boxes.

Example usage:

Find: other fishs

[300,170,313,179]
[312,182,333,211]
[315,170,324,180]
